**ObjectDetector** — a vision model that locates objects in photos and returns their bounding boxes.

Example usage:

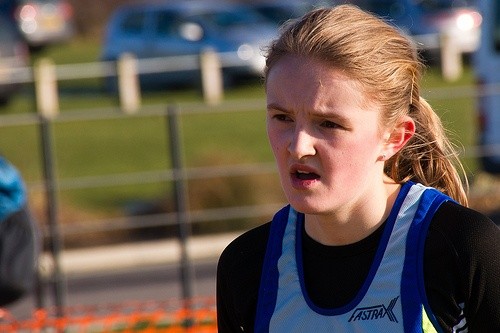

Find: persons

[215,4,500,333]
[0,156,40,315]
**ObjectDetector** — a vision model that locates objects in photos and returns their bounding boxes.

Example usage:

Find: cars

[100,3,282,93]
